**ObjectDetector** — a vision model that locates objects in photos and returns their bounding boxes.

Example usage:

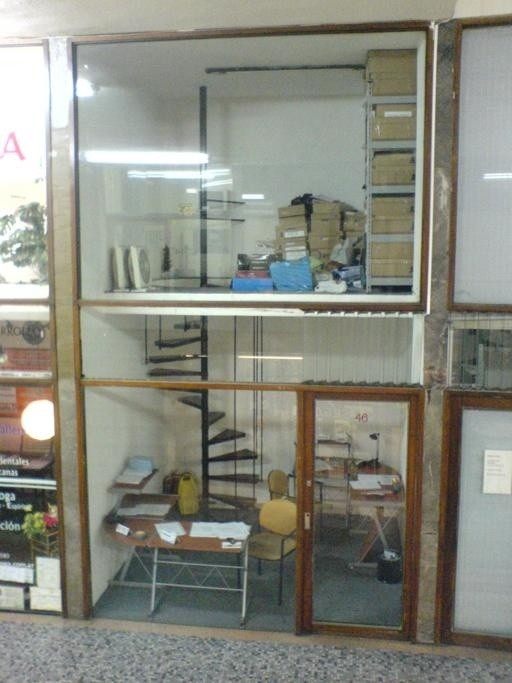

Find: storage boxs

[275,48,417,278]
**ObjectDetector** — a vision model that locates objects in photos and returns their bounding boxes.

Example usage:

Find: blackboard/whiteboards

[447,13,512,314]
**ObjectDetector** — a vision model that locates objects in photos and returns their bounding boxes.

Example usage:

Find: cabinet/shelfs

[365,80,416,294]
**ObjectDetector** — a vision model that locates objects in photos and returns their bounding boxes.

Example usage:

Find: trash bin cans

[376,548,402,584]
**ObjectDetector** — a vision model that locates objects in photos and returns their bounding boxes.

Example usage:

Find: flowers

[21,502,58,540]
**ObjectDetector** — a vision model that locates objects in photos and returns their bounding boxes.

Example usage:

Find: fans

[129,245,160,293]
[112,244,132,292]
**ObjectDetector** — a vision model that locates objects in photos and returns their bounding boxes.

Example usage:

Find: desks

[102,468,250,625]
[295,440,405,580]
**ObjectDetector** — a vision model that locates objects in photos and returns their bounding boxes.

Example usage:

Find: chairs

[268,470,296,503]
[237,498,298,606]
